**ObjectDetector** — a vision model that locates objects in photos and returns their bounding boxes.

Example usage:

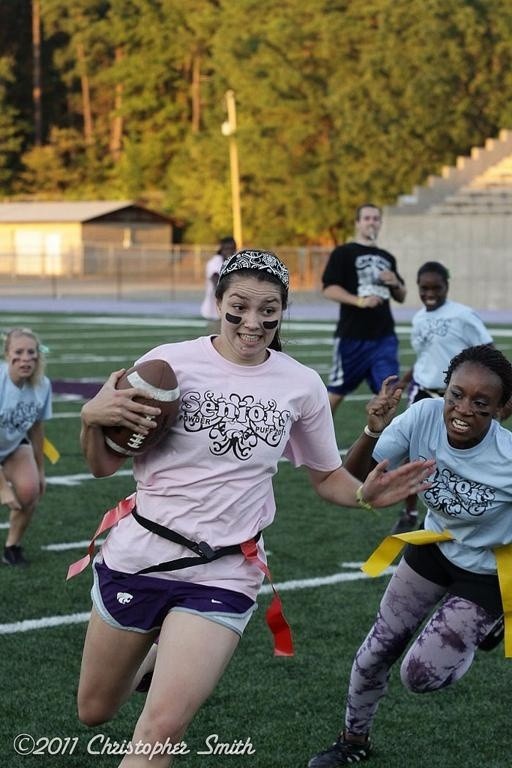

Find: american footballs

[100,360,180,457]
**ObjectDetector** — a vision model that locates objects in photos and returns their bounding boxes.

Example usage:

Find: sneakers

[478,617,506,651]
[307,732,372,767]
[3,544,28,566]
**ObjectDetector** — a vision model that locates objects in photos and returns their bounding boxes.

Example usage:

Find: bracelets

[355,484,381,517]
[357,297,364,308]
[363,423,382,439]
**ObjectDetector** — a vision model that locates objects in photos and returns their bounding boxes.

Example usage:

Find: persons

[320,203,408,417]
[66,249,439,768]
[0,328,54,572]
[389,260,497,535]
[305,344,512,768]
[199,236,236,336]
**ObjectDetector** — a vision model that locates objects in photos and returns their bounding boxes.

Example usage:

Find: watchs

[391,281,402,290]
